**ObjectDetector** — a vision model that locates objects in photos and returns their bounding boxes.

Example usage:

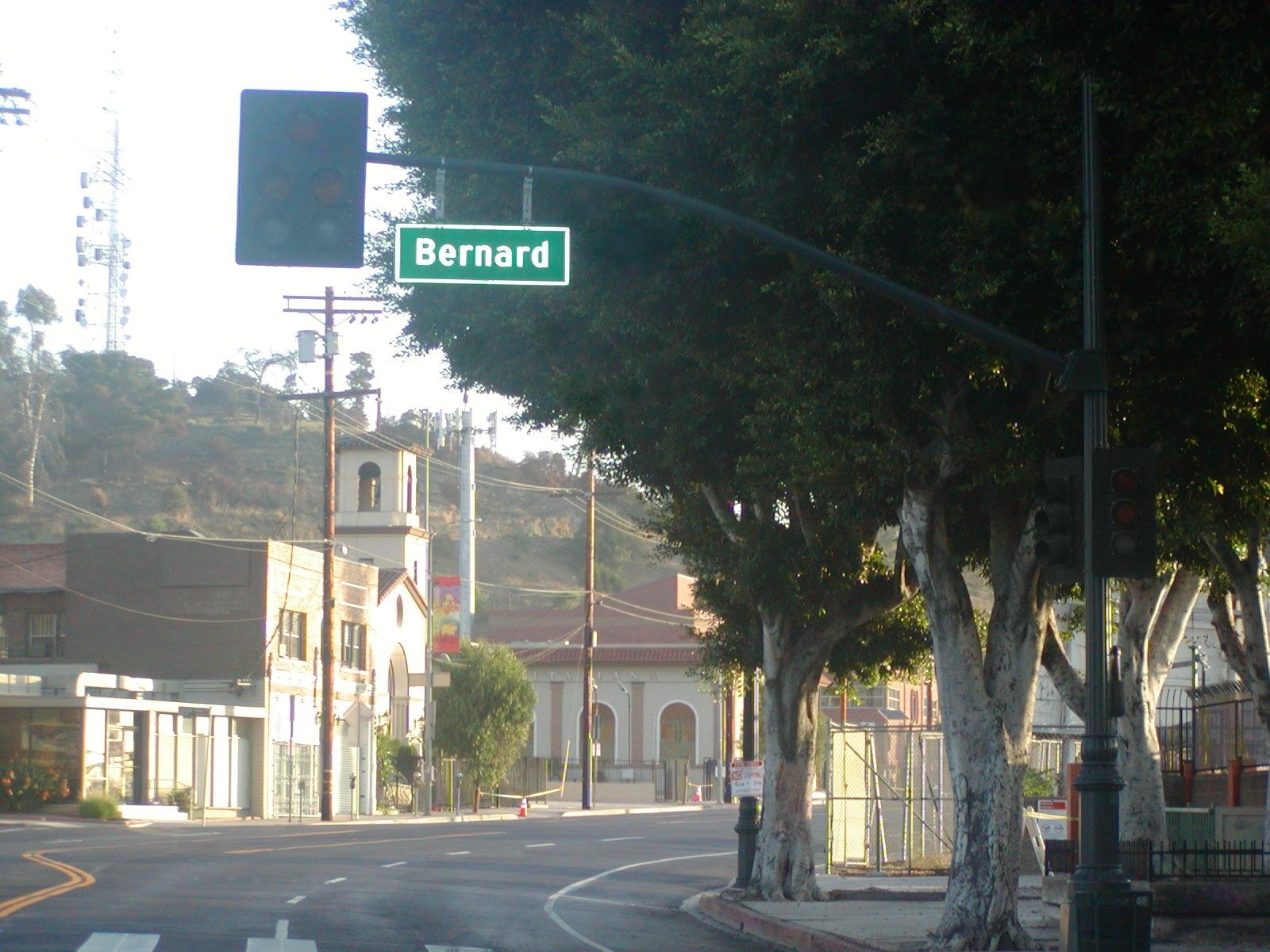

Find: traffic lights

[1111,468,1141,560]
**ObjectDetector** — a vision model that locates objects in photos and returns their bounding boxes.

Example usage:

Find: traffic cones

[695,785,703,803]
[515,793,529,817]
